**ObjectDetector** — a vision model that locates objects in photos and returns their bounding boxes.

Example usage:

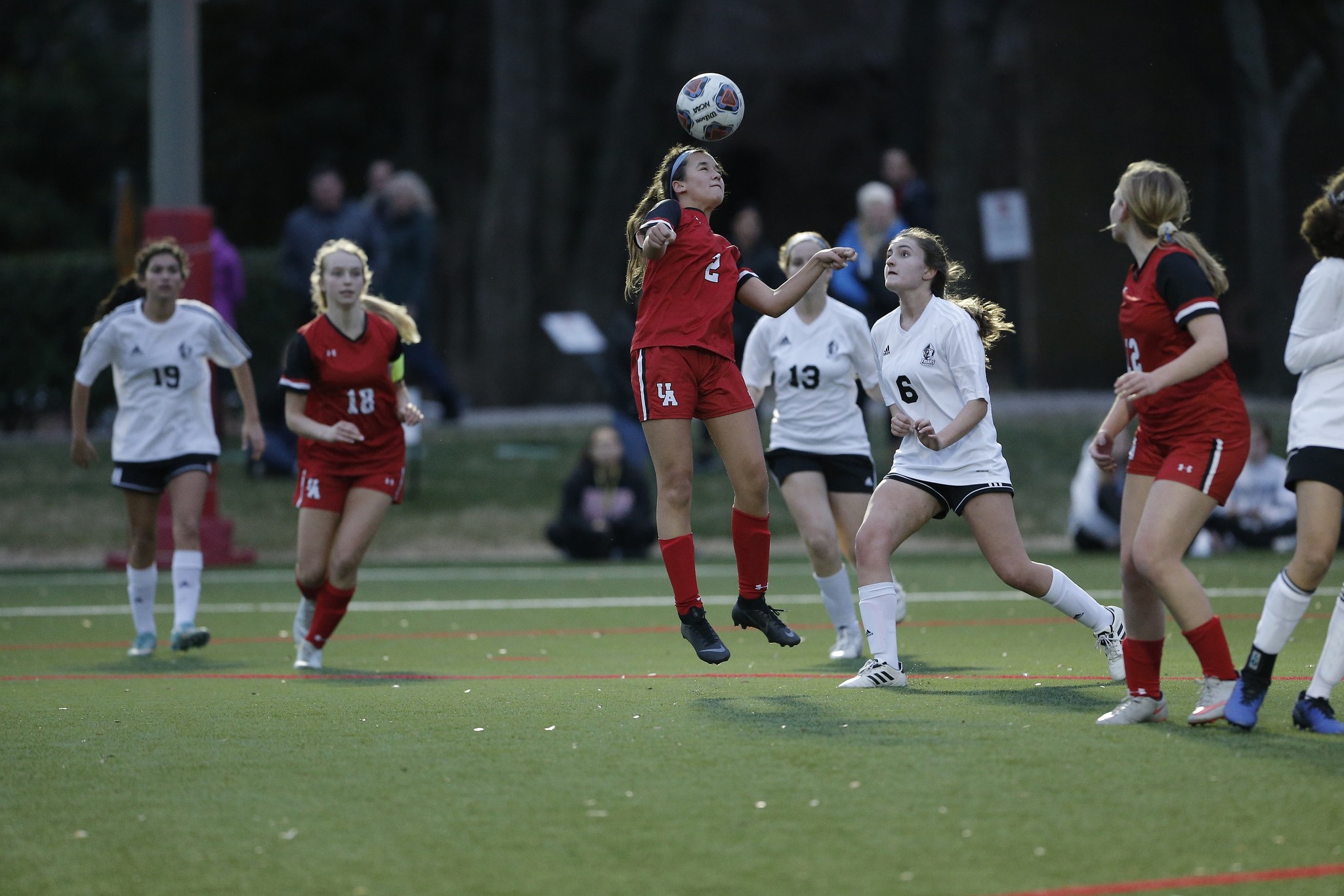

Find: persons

[1197,411,1298,559]
[232,155,471,502]
[883,146,937,241]
[1091,157,1253,730]
[735,233,906,663]
[602,290,665,508]
[819,182,918,307]
[276,235,427,671]
[1066,416,1142,562]
[623,146,863,666]
[694,208,790,471]
[543,425,660,558]
[836,226,1135,690]
[68,241,266,660]
[199,220,251,453]
[1221,171,1344,738]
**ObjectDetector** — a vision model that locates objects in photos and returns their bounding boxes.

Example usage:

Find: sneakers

[128,633,155,657]
[1223,676,1271,729]
[734,597,799,647]
[889,569,906,621]
[291,593,316,642]
[833,658,909,688]
[1097,688,1170,725]
[1093,604,1130,681]
[829,621,864,661]
[291,636,322,670]
[172,627,208,652]
[679,604,732,663]
[1183,667,1242,725]
[1292,690,1344,735]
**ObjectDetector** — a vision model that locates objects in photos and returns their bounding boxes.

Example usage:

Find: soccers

[676,71,748,144]
